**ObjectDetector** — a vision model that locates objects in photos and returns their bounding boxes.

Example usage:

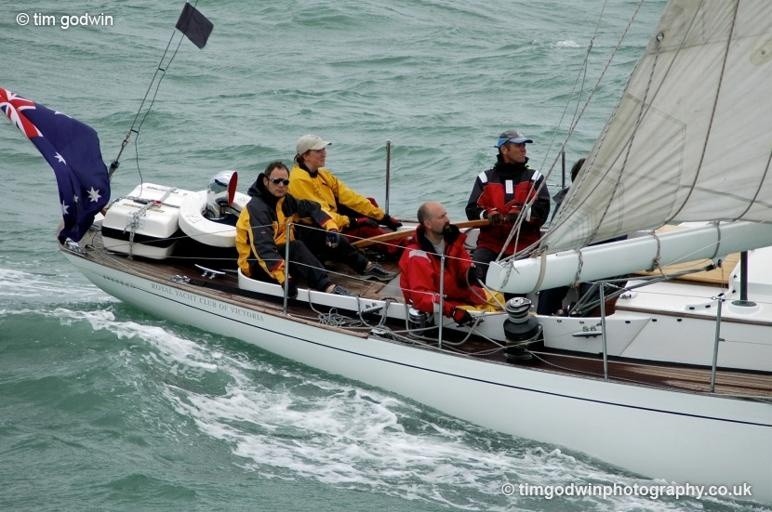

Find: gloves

[510,206,521,221]
[451,309,473,325]
[381,216,402,231]
[283,279,298,298]
[488,207,502,222]
[325,230,339,247]
[471,266,483,280]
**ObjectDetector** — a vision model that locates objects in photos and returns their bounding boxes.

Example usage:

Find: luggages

[100,181,194,261]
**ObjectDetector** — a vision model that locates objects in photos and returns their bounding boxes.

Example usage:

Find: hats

[296,134,333,156]
[498,130,533,146]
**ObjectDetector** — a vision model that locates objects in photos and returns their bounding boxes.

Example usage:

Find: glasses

[264,174,289,185]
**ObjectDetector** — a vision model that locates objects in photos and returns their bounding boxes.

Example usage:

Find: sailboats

[52,0,771,508]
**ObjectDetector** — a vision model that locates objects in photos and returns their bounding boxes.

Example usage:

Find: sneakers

[362,263,395,282]
[330,284,360,299]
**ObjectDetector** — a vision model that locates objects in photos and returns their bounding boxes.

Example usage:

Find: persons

[535,160,623,316]
[395,202,504,330]
[235,163,398,307]
[286,134,409,260]
[461,130,550,275]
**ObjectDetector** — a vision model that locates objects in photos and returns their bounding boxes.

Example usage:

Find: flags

[0,85,122,242]
